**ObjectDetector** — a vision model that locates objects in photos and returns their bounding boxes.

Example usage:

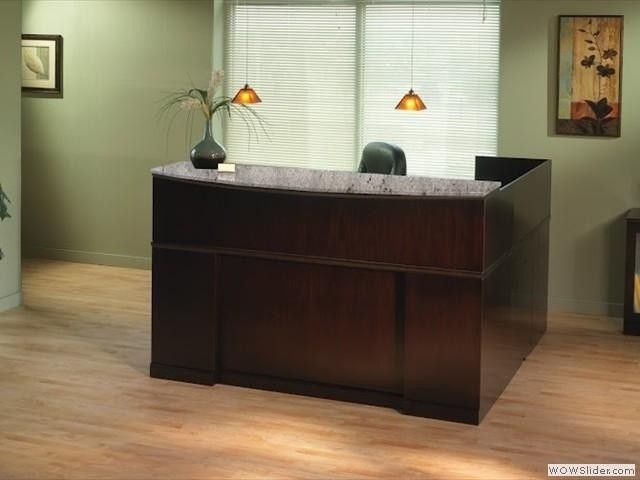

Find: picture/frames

[554,15,625,137]
[20,34,63,94]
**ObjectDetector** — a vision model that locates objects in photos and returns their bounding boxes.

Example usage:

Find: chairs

[356,141,407,177]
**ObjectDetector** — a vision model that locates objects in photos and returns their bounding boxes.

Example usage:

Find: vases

[188,118,227,170]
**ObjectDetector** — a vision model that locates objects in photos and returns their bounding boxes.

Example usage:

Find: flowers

[156,64,273,170]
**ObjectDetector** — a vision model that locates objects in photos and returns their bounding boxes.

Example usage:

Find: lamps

[229,0,263,105]
[395,0,427,111]
